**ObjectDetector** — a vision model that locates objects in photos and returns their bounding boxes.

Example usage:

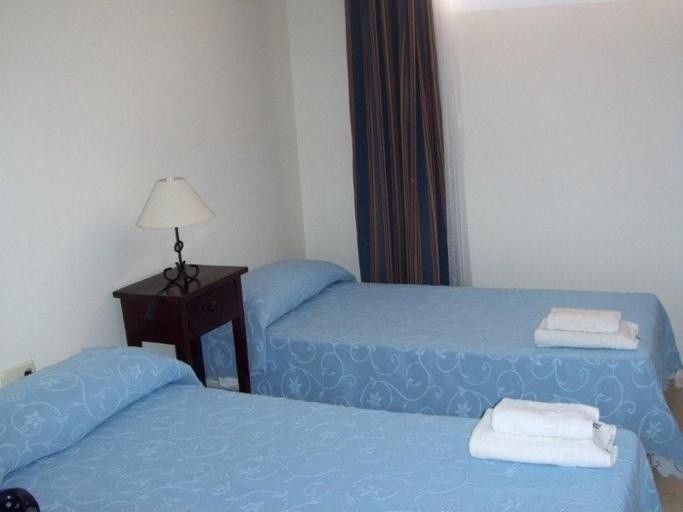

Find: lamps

[137,177,216,286]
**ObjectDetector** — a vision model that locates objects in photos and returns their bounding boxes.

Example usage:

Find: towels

[533,317,641,352]
[489,395,600,439]
[546,305,620,334]
[467,408,621,468]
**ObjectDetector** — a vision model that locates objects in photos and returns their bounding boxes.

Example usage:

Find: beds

[0,341,671,511]
[193,258,682,480]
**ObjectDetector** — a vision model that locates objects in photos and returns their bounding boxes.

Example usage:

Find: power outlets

[0,359,38,386]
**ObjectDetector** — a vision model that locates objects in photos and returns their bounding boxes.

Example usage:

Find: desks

[111,261,251,395]
